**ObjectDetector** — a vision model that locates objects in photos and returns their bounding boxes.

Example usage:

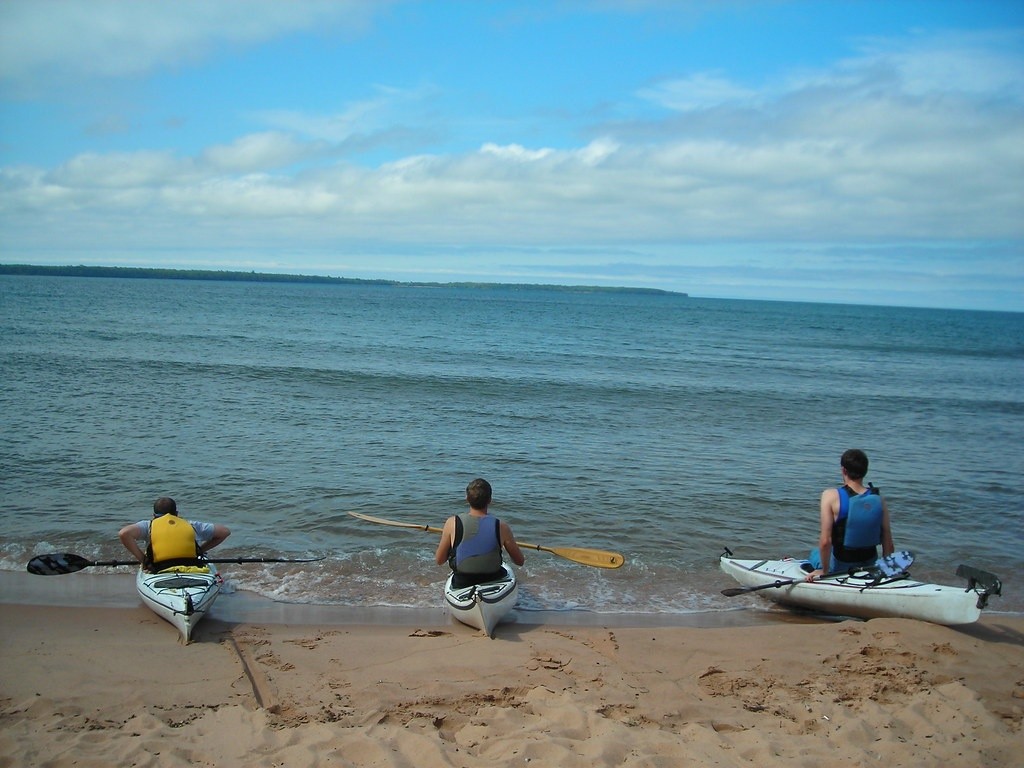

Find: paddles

[26,552,327,578]
[721,549,915,599]
[348,509,626,570]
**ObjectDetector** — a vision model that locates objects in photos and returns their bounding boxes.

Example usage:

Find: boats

[718,557,1001,626]
[444,561,518,638]
[136,548,224,642]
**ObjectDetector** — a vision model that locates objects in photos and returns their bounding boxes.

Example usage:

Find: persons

[435,478,524,588]
[118,497,231,571]
[804,448,894,581]
